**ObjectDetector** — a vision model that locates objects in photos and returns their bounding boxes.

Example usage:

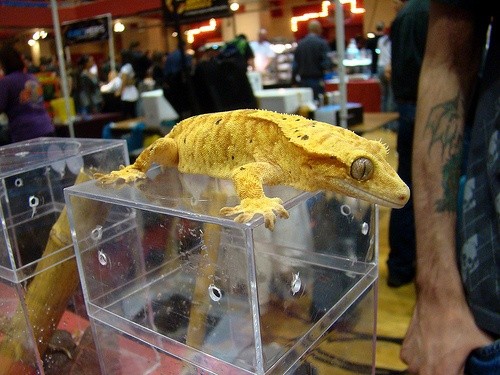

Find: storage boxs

[1,137,379,375]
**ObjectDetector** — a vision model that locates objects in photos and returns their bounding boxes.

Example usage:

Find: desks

[109,112,398,132]
[140,88,314,112]
[325,79,381,113]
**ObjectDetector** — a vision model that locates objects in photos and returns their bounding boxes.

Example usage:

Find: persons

[399,0,500,375]
[0,0,418,287]
